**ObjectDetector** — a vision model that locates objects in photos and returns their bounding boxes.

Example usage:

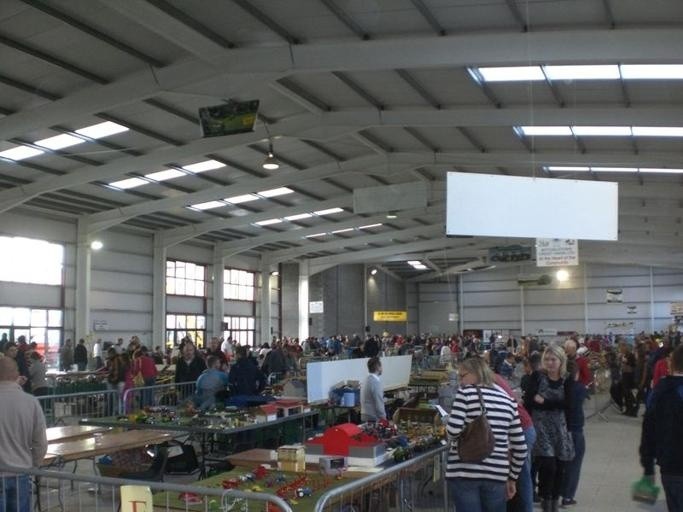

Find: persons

[1,357,48,511]
[2,333,48,411]
[562,329,683,417]
[444,356,527,510]
[521,344,588,511]
[639,347,682,512]
[360,357,386,424]
[490,372,537,512]
[60,335,305,450]
[423,333,548,375]
[304,332,422,358]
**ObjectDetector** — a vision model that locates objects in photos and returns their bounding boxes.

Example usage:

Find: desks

[40,401,324,492]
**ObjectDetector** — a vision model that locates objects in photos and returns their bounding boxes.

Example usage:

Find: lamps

[261,138,280,173]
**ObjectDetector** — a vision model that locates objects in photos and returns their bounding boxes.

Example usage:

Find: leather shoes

[534,491,576,511]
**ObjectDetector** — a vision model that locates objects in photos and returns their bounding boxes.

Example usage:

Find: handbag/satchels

[132,368,144,387]
[94,439,199,477]
[457,414,495,462]
